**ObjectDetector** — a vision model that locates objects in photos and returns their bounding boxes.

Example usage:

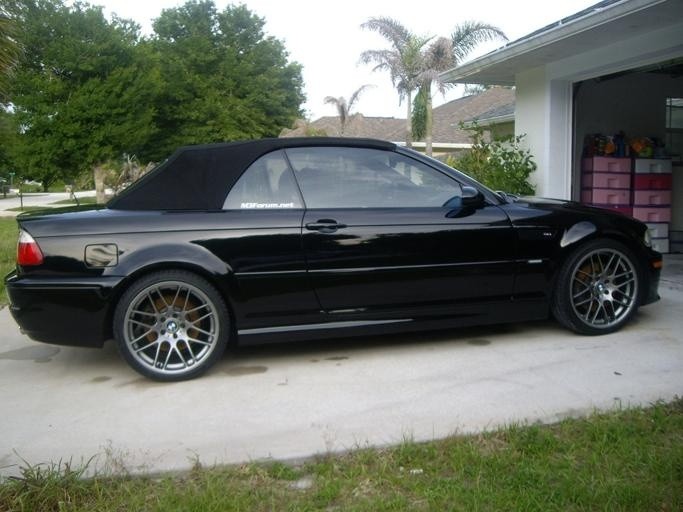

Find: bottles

[614,129,624,158]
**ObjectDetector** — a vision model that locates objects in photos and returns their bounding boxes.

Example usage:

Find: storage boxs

[582,154,671,256]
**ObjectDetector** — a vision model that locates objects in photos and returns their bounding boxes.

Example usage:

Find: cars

[3,136,664,381]
